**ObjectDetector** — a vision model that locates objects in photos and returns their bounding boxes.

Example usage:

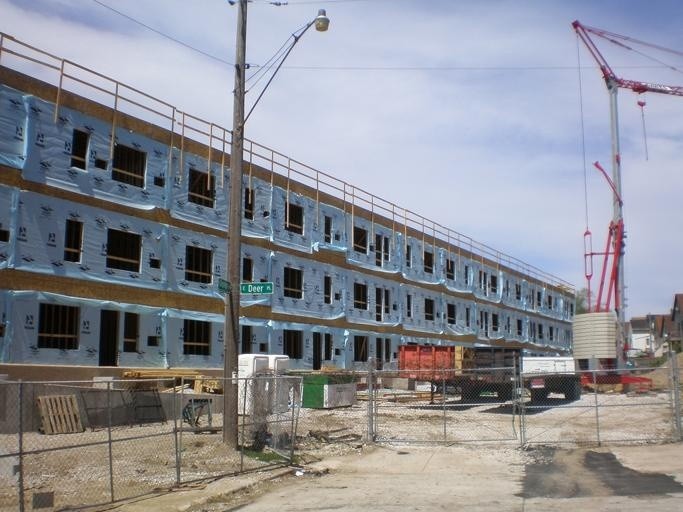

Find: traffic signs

[239,282,276,295]
[218,280,231,294]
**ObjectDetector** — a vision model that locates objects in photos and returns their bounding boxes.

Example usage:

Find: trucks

[399,338,582,404]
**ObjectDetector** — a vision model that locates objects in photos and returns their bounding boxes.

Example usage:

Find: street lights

[222,0,331,451]
